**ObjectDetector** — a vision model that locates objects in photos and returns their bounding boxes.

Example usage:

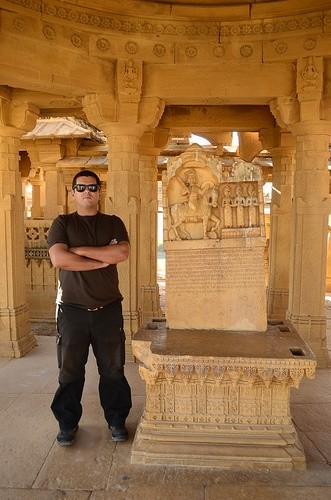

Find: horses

[169,189,221,241]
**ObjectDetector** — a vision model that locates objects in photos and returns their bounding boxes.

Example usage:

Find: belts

[63,299,120,312]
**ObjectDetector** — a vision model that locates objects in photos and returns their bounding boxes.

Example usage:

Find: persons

[48,170,132,446]
[246,185,259,227]
[222,185,234,228]
[182,170,210,222]
[234,184,246,228]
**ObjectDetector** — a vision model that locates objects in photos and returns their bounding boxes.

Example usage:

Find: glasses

[73,184,100,192]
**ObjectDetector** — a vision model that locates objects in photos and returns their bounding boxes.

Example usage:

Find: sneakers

[56,425,78,445]
[110,425,127,441]
